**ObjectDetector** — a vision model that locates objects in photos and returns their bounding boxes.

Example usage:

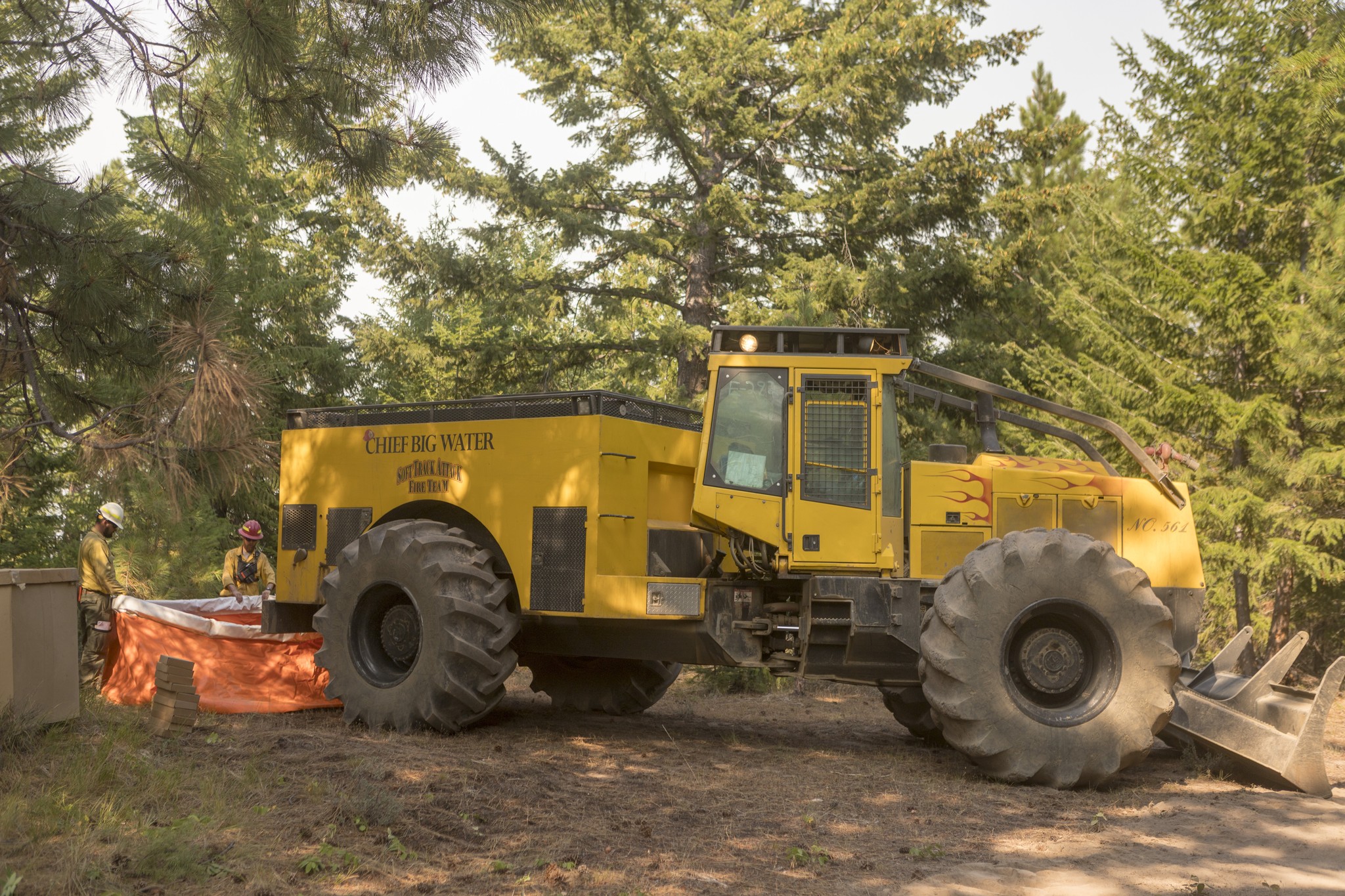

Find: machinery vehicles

[262,323,1344,802]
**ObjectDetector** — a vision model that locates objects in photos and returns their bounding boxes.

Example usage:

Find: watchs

[265,587,271,592]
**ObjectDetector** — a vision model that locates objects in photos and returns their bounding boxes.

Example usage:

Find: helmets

[96,502,124,530]
[238,520,263,539]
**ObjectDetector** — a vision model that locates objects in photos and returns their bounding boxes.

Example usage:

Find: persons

[220,519,276,604]
[77,501,145,702]
